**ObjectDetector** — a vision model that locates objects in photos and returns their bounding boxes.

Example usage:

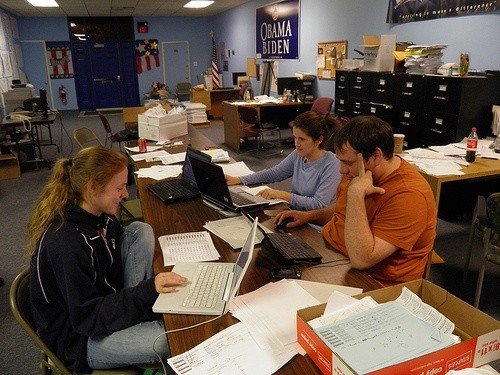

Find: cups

[393,134,405,154]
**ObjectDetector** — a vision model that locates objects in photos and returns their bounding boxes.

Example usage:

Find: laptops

[238,208,322,264]
[145,146,269,213]
[153,217,258,315]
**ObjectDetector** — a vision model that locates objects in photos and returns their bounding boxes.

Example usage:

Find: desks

[124,122,384,375]
[0,110,62,170]
[189,88,251,119]
[224,99,316,152]
[393,138,500,281]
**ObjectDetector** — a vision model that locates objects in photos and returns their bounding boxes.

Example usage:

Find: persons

[273,115,437,283]
[26,146,188,375]
[223,111,347,233]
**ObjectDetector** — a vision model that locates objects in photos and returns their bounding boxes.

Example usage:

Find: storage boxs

[297,278,500,375]
[360,34,395,72]
[138,109,188,142]
[0,147,20,180]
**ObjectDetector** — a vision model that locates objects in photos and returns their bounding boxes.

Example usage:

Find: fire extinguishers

[59,85,67,103]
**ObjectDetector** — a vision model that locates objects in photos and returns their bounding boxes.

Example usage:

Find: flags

[211,37,221,89]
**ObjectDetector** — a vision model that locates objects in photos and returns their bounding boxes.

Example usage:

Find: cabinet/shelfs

[335,70,500,222]
[198,73,223,89]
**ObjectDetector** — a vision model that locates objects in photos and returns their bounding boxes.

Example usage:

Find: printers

[2,84,34,117]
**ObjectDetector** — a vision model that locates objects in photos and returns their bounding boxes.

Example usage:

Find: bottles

[246,91,250,102]
[466,127,479,163]
[282,88,300,103]
[460,54,469,77]
[449,66,452,76]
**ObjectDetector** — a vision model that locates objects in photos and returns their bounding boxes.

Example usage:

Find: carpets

[77,109,123,117]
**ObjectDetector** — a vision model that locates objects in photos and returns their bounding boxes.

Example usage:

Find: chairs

[115,197,144,225]
[73,127,104,149]
[237,106,283,159]
[289,98,334,133]
[97,111,130,151]
[9,266,172,375]
[13,98,49,144]
[464,193,500,308]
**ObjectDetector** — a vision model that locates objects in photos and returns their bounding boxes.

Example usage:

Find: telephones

[10,114,32,121]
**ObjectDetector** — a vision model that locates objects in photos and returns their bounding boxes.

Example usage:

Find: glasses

[339,159,356,168]
